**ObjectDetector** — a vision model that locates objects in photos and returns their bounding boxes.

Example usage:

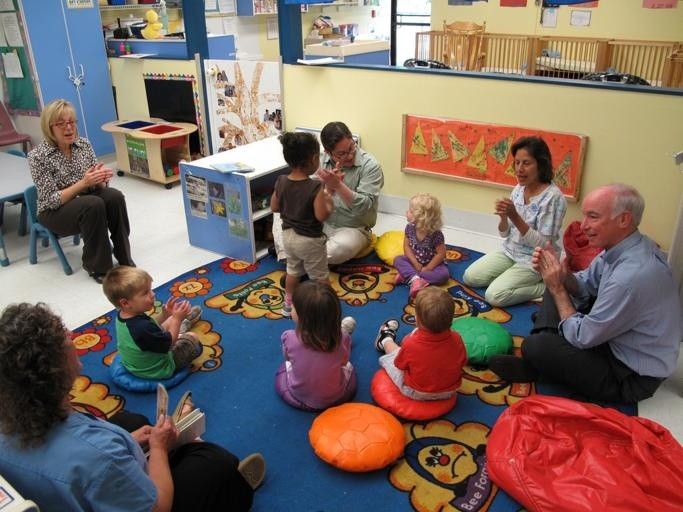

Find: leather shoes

[83,263,105,284]
[488,355,539,383]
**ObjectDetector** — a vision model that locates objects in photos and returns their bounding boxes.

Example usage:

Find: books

[208,158,255,175]
[141,408,210,458]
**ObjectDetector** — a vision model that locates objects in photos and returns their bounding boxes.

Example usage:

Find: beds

[415,31,683,89]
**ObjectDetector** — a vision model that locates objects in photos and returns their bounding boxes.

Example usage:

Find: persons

[27,98,137,285]
[0,301,266,512]
[374,284,468,402]
[280,280,357,410]
[462,135,567,309]
[102,264,203,380]
[391,193,450,297]
[269,130,335,320]
[268,121,385,270]
[487,183,682,405]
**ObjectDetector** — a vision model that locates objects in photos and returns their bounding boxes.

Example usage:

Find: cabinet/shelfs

[178,126,361,266]
[97,0,391,67]
[100,118,198,189]
[0,0,118,167]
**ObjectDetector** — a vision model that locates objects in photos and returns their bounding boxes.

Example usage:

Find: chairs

[0,101,114,275]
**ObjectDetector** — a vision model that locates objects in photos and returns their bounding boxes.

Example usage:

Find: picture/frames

[400,112,590,204]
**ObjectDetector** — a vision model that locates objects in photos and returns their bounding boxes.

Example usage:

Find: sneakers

[179,318,191,333]
[410,279,429,299]
[281,302,292,317]
[394,274,404,285]
[340,316,356,335]
[374,318,400,351]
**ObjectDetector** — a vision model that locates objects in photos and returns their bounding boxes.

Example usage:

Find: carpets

[66,235,640,511]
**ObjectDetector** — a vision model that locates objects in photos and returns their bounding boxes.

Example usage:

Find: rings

[543,263,549,270]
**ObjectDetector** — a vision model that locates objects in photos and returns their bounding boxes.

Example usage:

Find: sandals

[238,454,265,489]
[172,391,193,422]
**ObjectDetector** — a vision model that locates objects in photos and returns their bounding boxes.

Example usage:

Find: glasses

[331,144,356,158]
[51,120,78,127]
